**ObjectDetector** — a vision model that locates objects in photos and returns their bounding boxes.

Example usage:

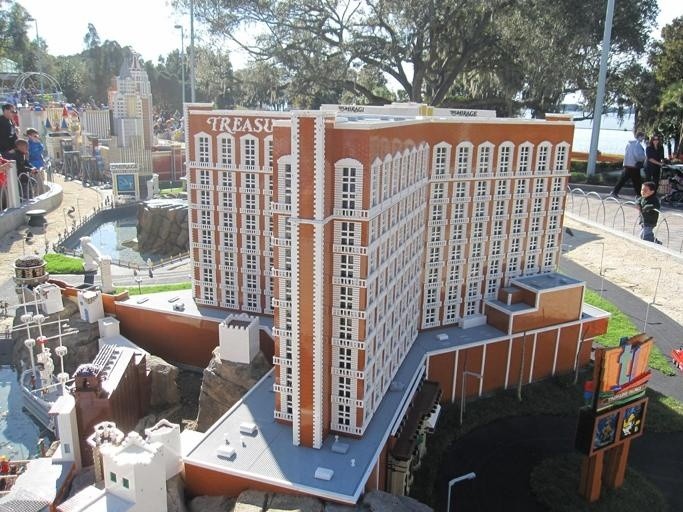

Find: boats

[2,284,77,441]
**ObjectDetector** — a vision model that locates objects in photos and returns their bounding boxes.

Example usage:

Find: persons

[644,134,665,193]
[0,104,43,200]
[152,104,183,136]
[635,181,662,245]
[610,132,645,199]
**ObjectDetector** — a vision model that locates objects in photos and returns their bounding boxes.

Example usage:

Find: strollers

[659,163,682,207]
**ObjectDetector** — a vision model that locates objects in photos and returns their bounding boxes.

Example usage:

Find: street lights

[20,235,36,257]
[27,17,43,96]
[649,266,661,303]
[459,370,481,424]
[590,241,605,275]
[598,267,617,299]
[61,206,71,234]
[174,25,184,116]
[75,197,85,220]
[643,301,663,334]
[444,472,476,512]
[42,220,56,251]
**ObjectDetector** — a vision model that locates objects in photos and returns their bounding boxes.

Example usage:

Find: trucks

[671,345,682,373]
